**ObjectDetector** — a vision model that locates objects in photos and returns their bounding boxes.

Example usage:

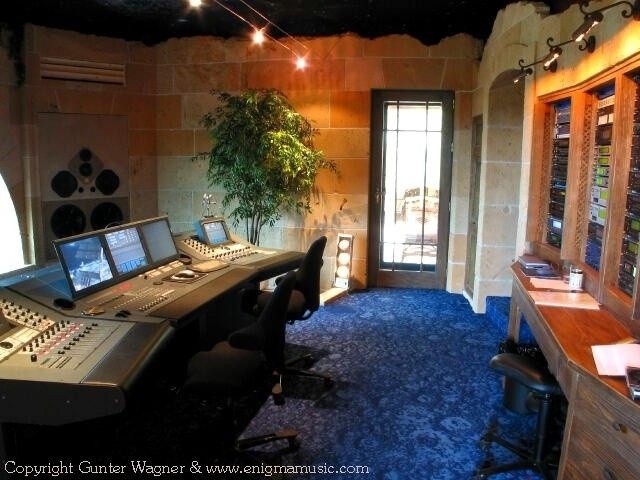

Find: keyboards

[189,258,229,273]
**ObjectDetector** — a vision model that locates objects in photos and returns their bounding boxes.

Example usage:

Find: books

[519,268,561,278]
[528,291,603,310]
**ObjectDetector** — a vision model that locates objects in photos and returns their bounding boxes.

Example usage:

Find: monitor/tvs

[197,218,233,245]
[52,215,181,299]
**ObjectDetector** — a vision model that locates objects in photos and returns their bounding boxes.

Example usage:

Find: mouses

[175,269,195,277]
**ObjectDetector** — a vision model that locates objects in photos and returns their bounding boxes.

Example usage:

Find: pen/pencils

[546,290,579,293]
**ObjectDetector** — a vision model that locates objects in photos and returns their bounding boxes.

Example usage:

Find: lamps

[512,0,640,85]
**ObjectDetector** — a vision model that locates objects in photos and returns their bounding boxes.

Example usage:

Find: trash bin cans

[498,339,558,414]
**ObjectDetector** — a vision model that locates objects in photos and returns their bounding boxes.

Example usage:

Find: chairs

[188,236,334,450]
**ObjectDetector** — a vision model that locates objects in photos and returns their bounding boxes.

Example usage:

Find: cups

[569,266,583,291]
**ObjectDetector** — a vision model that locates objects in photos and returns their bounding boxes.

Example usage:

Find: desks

[507,255,640,480]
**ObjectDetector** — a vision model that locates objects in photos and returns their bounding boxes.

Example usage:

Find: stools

[477,351,564,480]
